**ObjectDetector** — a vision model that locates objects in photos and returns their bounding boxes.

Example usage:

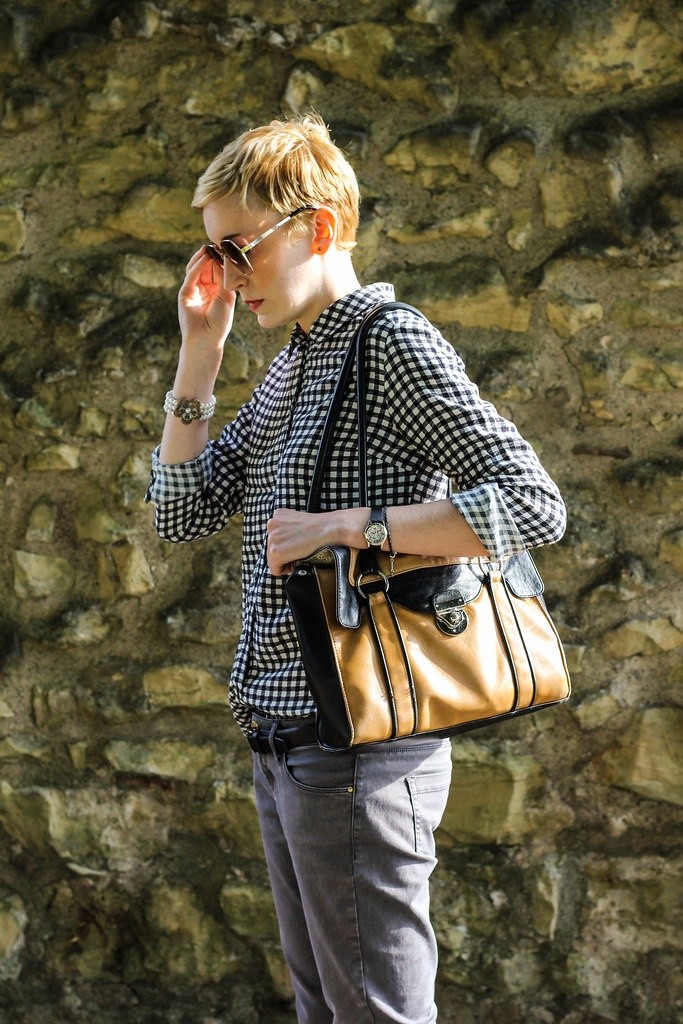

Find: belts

[247,722,319,755]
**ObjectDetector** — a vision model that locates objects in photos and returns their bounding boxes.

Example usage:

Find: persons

[143,117,563,1024]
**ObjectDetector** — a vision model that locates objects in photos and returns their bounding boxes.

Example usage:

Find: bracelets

[383,504,396,573]
[163,390,216,424]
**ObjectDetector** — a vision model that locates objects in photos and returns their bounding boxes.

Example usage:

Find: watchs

[364,506,387,552]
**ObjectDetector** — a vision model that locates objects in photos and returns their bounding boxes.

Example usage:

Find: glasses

[205,206,318,276]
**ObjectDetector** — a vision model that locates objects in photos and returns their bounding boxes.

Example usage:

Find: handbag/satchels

[282,300,571,752]
